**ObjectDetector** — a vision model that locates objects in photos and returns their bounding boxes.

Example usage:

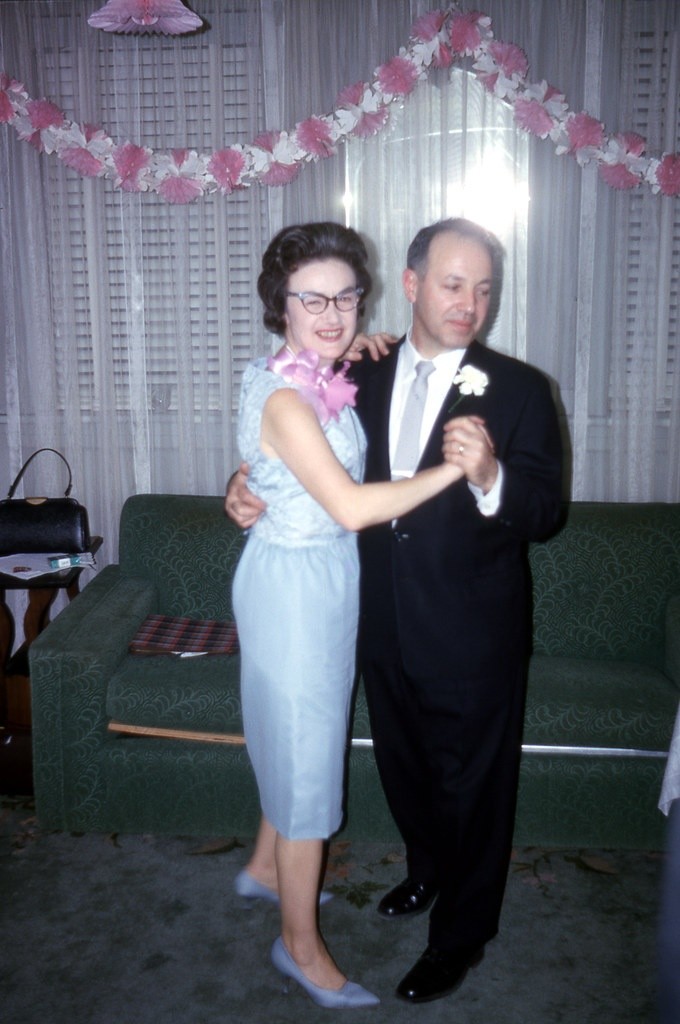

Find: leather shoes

[395,938,485,1004]
[376,874,436,921]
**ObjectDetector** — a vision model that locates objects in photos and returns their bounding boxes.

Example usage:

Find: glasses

[281,283,366,314]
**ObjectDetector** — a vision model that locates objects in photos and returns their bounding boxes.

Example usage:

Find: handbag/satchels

[0,447,91,555]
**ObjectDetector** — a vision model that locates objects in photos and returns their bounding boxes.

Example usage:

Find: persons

[231,221,494,1010]
[658,703,680,1024]
[224,215,572,1004]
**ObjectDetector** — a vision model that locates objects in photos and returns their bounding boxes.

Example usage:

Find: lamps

[87,0,204,36]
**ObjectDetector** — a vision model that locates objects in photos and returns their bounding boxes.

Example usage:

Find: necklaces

[285,343,300,358]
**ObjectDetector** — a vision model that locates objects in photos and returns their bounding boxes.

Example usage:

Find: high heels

[268,935,383,1008]
[232,866,336,910]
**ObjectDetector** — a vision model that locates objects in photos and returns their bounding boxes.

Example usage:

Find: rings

[459,445,465,455]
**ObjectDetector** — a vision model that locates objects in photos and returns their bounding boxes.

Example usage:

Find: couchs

[26,495,680,851]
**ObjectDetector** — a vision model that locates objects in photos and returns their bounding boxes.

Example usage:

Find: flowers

[448,365,488,414]
[0,0,680,207]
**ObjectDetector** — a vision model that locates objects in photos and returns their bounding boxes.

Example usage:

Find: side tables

[0,536,104,795]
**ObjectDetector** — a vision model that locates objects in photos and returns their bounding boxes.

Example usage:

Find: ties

[392,361,434,485]
